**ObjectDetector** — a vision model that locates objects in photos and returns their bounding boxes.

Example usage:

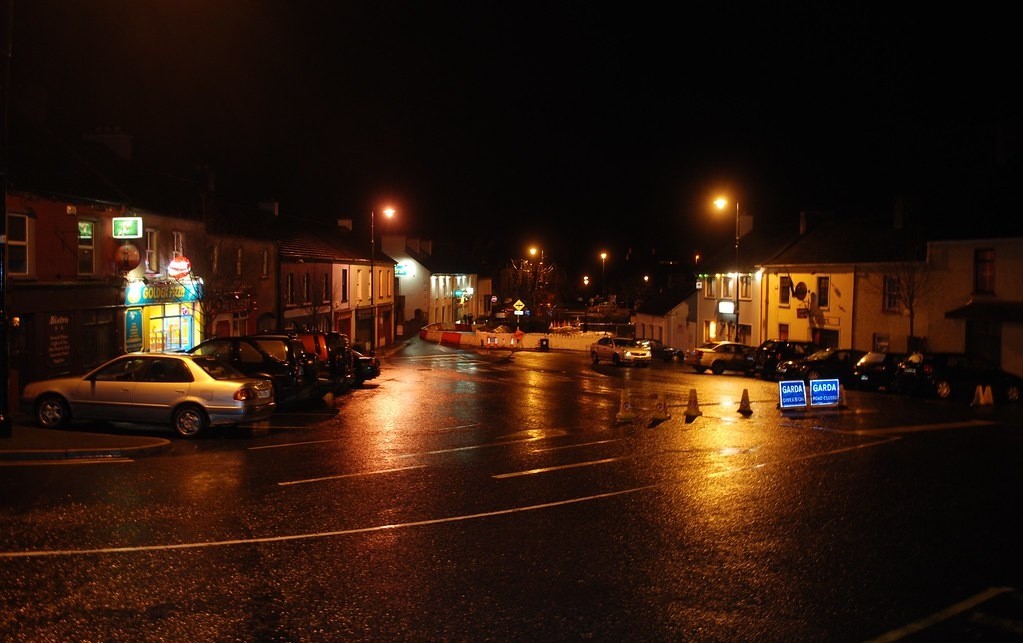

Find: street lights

[601,253,607,289]
[583,277,588,332]
[712,194,740,342]
[369,207,396,356]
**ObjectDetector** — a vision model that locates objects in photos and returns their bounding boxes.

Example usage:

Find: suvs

[189,331,381,405]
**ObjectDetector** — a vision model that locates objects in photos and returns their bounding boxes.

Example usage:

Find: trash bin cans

[540,339,549,351]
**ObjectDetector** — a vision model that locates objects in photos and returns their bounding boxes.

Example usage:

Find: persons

[463,314,467,324]
[468,312,473,324]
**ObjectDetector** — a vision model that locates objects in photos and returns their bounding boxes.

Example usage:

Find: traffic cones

[971,386,994,407]
[684,389,703,415]
[650,391,671,420]
[737,389,753,414]
[615,390,637,418]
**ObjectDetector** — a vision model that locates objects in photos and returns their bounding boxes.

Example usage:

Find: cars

[635,338,685,364]
[776,346,866,385]
[22,352,277,439]
[590,337,652,367]
[754,338,820,374]
[684,342,757,375]
[852,350,1023,402]
